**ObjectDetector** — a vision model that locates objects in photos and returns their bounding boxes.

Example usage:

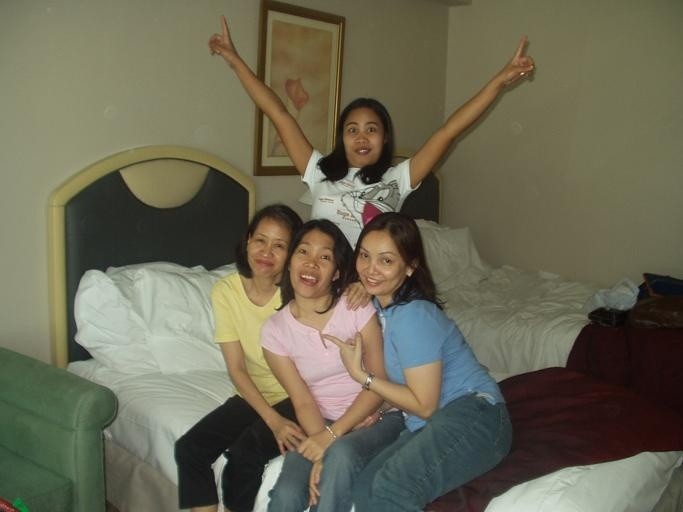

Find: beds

[50,145,682,509]
[384,156,682,408]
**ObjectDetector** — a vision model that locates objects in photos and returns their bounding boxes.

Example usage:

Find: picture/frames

[252,1,346,177]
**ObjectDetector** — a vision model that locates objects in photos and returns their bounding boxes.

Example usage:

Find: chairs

[0,345,119,511]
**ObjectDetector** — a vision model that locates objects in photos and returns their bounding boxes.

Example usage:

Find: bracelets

[377,406,385,423]
[324,424,338,442]
[360,373,375,391]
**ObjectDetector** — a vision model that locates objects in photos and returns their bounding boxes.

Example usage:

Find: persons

[207,13,537,253]
[256,218,387,512]
[320,212,514,512]
[172,202,308,512]
